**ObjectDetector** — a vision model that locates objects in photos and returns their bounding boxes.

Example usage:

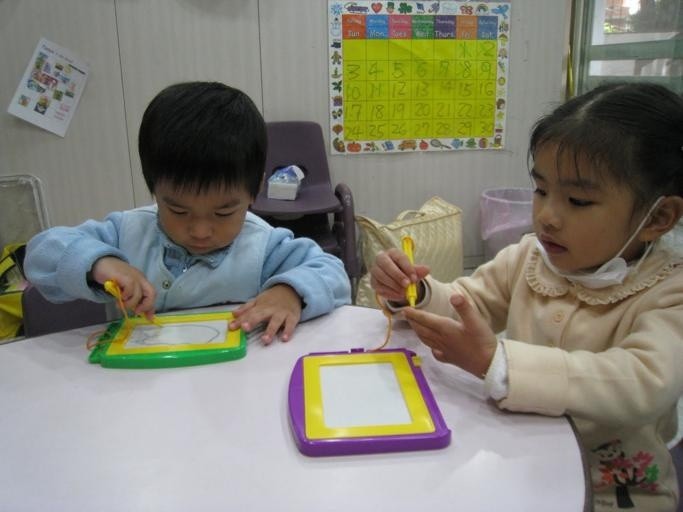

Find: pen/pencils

[401,238,417,310]
[104,279,156,323]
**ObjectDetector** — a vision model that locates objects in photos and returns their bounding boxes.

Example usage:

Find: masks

[535,194,665,288]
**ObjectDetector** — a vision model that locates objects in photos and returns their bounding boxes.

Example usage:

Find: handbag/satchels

[354,194,463,309]
[0,242,30,341]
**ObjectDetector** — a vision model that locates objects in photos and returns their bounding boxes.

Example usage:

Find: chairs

[0,174,49,245]
[21,282,108,340]
[248,120,359,280]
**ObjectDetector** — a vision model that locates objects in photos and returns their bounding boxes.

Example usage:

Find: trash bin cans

[479,189,533,262]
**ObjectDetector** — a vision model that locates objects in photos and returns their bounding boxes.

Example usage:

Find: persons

[368,81,683,512]
[21,81,355,347]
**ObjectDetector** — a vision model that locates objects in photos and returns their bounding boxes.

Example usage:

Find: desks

[0,302,594,512]
